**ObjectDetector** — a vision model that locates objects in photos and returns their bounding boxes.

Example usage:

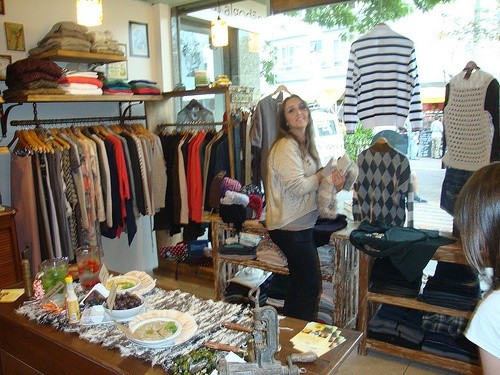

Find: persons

[452,163,500,375]
[431,116,443,159]
[405,117,419,160]
[432,104,440,110]
[263,95,346,322]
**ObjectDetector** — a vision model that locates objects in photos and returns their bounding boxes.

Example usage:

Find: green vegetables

[121,283,134,289]
[164,321,177,334]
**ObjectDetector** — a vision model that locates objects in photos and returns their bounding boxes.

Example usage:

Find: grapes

[112,293,141,310]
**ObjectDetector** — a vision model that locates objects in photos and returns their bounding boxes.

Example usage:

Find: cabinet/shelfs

[211,215,486,375]
[0,50,164,104]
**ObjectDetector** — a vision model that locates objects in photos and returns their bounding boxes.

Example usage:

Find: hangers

[466,61,479,70]
[178,100,212,116]
[12,114,145,153]
[270,84,292,97]
[377,132,388,144]
[230,92,257,117]
[157,121,227,142]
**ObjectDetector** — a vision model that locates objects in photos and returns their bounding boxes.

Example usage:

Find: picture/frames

[0,53,12,80]
[129,20,150,58]
[106,43,128,80]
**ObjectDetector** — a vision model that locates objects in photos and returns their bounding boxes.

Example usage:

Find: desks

[0,265,364,375]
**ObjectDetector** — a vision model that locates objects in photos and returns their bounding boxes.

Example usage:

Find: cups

[75,245,101,291]
[40,256,69,297]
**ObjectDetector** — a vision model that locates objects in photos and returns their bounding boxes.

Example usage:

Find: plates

[129,317,182,343]
[80,305,145,325]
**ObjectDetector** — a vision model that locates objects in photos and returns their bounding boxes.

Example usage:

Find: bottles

[64,274,81,328]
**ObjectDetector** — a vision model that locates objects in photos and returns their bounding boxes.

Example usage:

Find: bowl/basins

[106,276,141,295]
[102,295,145,318]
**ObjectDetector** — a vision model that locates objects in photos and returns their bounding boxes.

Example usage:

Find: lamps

[76,0,103,28]
[209,0,229,47]
